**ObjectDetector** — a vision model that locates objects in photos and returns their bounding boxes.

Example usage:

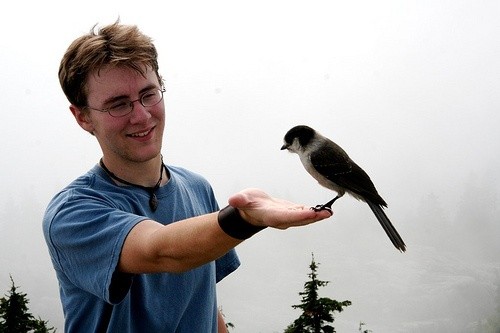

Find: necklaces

[100,154,166,214]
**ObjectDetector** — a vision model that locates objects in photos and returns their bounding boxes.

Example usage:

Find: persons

[41,16,333,333]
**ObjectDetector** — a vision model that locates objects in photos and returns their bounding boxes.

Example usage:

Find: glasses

[88,80,166,118]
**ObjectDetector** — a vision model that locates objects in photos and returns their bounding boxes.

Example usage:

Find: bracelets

[217,204,269,241]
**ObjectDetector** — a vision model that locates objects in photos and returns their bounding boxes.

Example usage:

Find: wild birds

[280,122,408,256]
